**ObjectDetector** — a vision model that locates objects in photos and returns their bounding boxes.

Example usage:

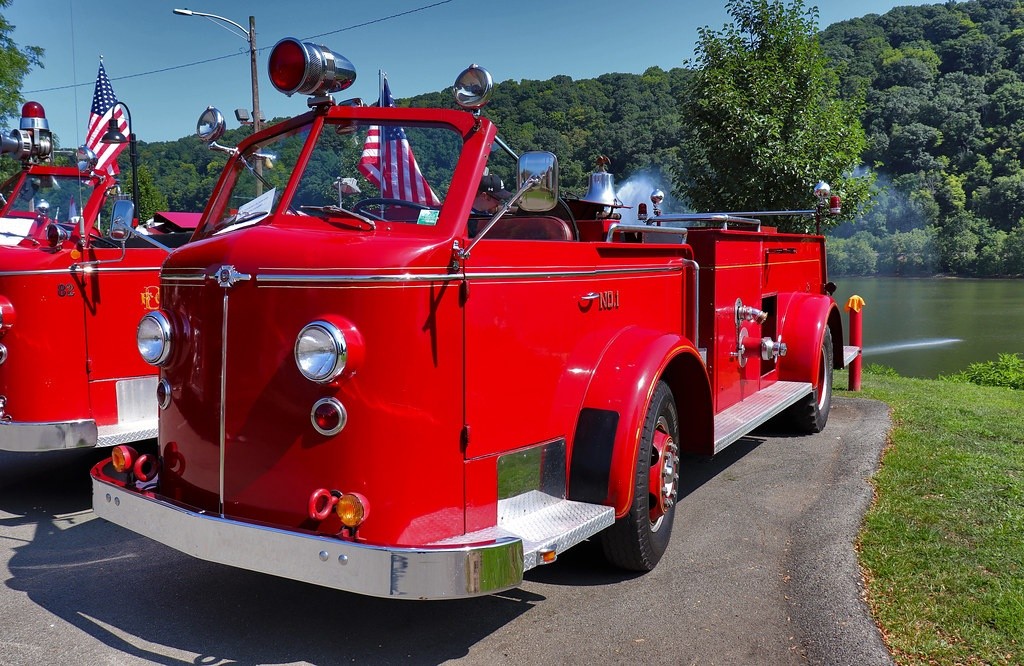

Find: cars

[0,100,243,454]
[87,36,862,601]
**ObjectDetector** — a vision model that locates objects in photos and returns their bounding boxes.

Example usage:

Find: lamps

[251,110,266,122]
[235,109,250,121]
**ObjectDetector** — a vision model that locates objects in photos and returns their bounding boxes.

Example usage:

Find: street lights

[171,6,265,198]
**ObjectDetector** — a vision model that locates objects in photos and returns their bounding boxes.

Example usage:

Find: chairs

[90,232,192,248]
[477,216,573,242]
[371,204,442,224]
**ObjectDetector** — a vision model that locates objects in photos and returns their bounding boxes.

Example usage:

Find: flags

[77,62,131,191]
[359,76,440,209]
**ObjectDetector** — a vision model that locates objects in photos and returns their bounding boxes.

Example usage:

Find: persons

[470,173,519,215]
